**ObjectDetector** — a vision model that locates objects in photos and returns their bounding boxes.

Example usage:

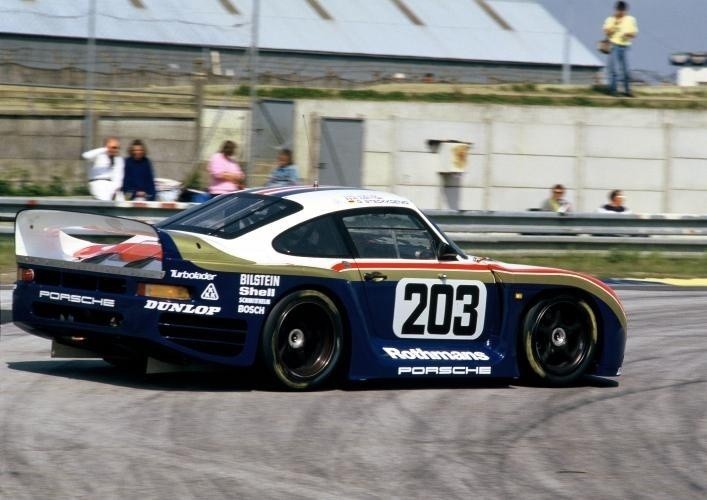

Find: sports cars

[9,179,629,394]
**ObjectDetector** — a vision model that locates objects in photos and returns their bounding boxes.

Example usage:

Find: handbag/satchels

[596,40,610,54]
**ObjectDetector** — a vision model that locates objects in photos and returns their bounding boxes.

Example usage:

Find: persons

[206,140,246,195]
[540,184,571,214]
[261,149,300,190]
[600,190,628,213]
[600,0,639,96]
[81,138,126,201]
[121,139,155,201]
